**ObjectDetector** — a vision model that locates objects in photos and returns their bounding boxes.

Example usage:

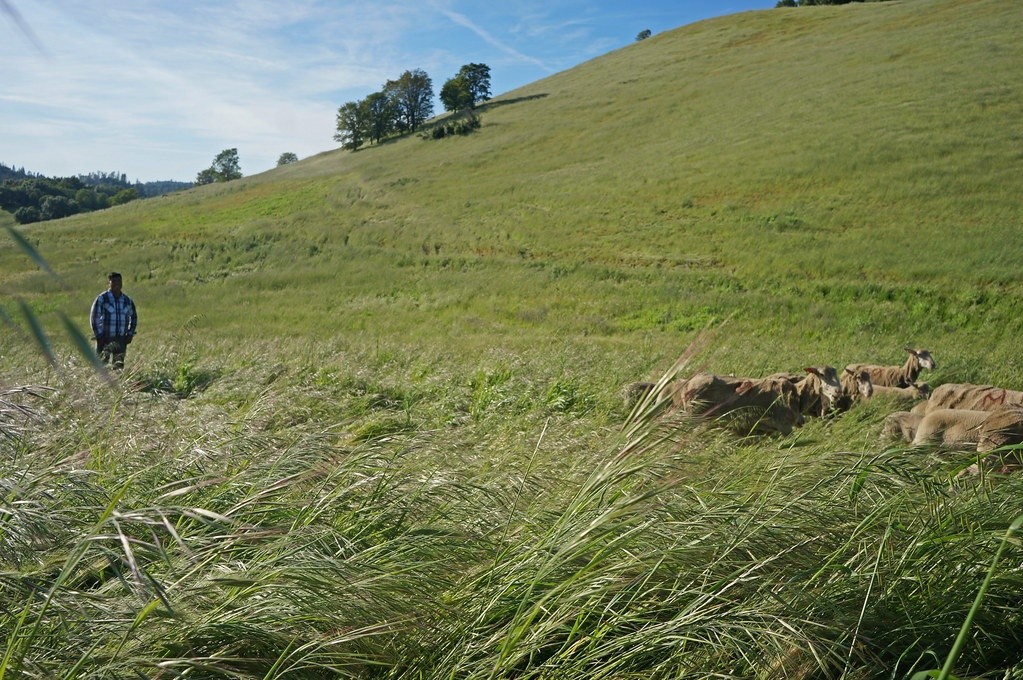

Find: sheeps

[624,356,871,437]
[857,380,935,405]
[842,347,935,389]
[882,384,1023,477]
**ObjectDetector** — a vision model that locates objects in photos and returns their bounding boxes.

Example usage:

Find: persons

[88,271,137,370]
